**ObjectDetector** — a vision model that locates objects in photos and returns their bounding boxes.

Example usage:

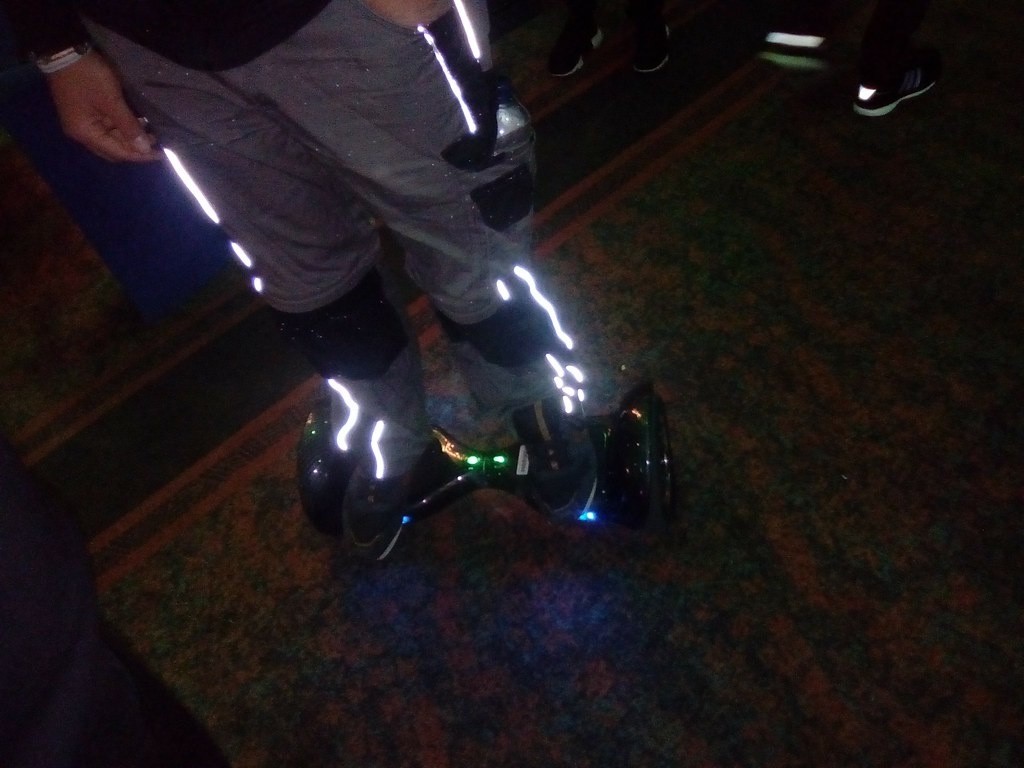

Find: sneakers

[852,64,936,117]
[343,437,441,561]
[766,43,829,70]
[506,397,598,524]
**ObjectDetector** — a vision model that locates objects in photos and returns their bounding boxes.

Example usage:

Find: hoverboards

[291,397,682,557]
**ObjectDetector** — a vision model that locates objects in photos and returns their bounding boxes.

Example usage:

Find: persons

[544,1,674,78]
[755,0,946,118]
[1,0,598,563]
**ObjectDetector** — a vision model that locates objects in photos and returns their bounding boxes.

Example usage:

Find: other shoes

[548,16,603,76]
[633,17,670,72]
